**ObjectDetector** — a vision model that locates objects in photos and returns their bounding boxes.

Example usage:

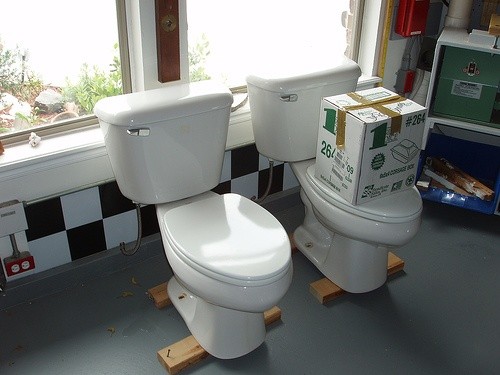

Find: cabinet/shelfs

[415,26,500,225]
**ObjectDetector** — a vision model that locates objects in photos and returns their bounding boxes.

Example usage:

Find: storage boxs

[316,86,427,206]
[435,46,500,124]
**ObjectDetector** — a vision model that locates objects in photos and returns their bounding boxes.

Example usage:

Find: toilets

[93,80,293,360]
[246,54,422,294]
